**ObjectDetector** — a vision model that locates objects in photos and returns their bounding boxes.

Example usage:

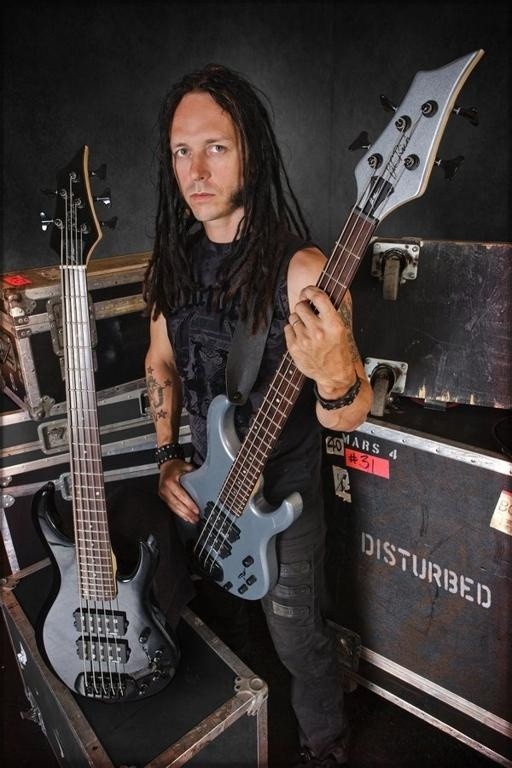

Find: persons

[143,63,375,768]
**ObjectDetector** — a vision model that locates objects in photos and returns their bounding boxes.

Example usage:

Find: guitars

[32,143,182,703]
[172,49,484,605]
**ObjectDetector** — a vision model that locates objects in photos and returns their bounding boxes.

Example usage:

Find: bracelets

[313,379,362,410]
[155,443,185,469]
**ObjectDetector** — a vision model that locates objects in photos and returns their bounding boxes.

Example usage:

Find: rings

[292,318,301,328]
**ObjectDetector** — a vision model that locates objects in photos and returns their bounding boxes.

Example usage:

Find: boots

[300,728,351,768]
